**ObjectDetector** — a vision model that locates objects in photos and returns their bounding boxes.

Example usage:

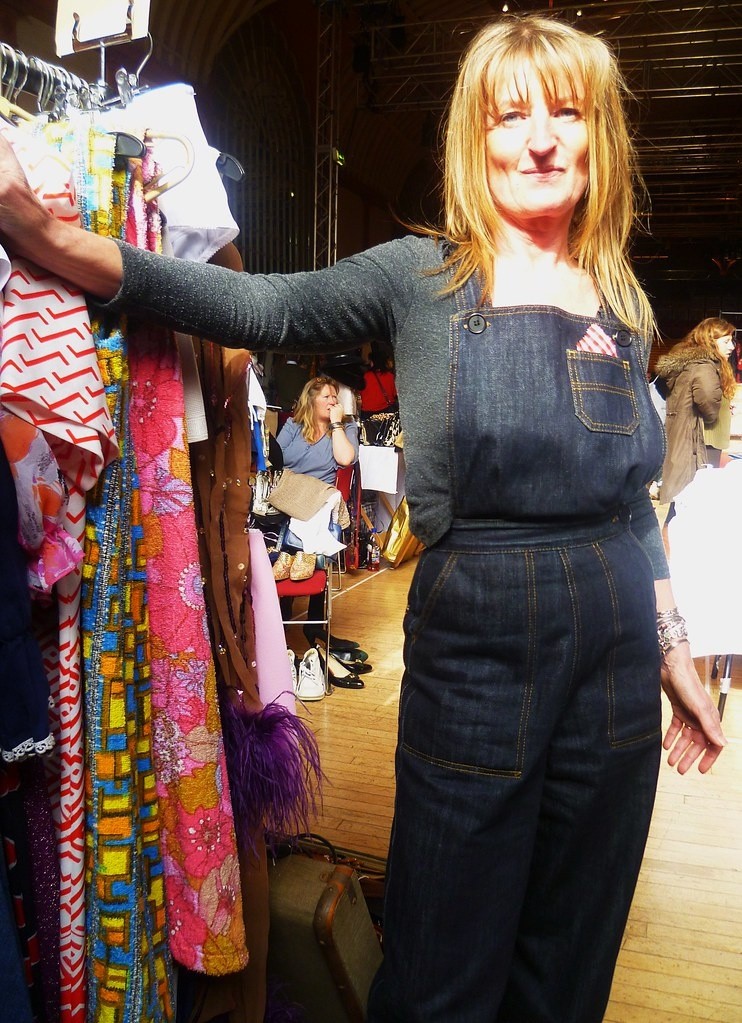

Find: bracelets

[656,607,690,658]
[329,422,345,432]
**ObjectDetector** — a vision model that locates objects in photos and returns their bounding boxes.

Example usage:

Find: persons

[276,376,360,625]
[648,375,669,500]
[361,351,396,418]
[0,13,727,1023]
[655,318,736,561]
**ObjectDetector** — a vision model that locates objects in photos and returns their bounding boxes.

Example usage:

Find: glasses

[307,377,338,394]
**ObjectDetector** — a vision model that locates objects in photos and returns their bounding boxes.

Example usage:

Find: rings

[685,725,692,730]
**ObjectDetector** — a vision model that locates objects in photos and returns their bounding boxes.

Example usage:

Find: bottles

[367,528,381,572]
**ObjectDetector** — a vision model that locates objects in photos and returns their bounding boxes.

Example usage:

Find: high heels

[303,623,372,689]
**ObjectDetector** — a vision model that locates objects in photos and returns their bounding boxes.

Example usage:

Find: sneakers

[298,647,326,700]
[287,649,297,694]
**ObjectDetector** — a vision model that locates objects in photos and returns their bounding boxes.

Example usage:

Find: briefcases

[263,828,389,1023]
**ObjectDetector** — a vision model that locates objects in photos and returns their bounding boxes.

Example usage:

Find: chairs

[275,465,353,695]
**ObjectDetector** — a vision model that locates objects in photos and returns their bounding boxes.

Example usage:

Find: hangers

[1,44,244,200]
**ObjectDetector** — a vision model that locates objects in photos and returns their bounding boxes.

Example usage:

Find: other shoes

[273,551,317,581]
